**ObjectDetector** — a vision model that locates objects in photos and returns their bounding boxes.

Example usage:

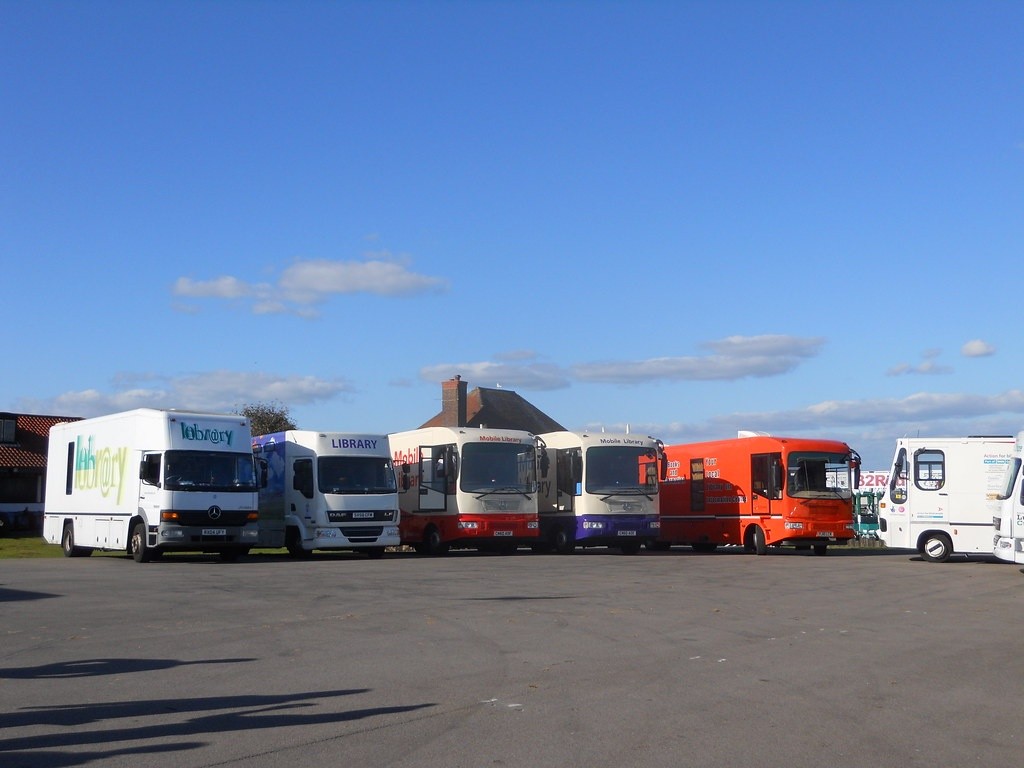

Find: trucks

[877,436,1017,565]
[387,426,551,557]
[520,423,667,556]
[238,430,401,561]
[42,408,269,564]
[638,430,861,556]
[991,430,1024,566]
